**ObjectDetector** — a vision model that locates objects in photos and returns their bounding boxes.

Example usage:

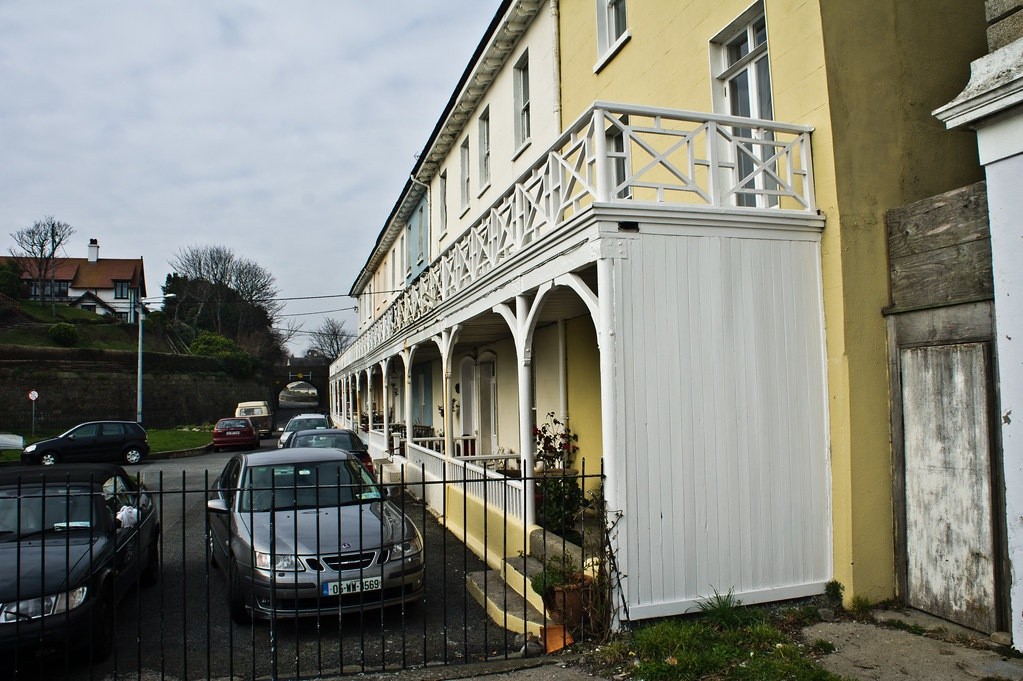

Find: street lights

[136,294,176,424]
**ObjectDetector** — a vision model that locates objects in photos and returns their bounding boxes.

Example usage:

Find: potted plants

[539,473,582,547]
[517,548,593,624]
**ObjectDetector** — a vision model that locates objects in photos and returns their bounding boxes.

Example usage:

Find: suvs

[277,412,338,448]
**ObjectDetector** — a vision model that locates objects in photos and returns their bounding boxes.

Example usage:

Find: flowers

[533,412,579,470]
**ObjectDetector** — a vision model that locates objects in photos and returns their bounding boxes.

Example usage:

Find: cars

[0,460,160,663]
[283,428,375,492]
[211,416,260,453]
[205,447,428,628]
[21,420,150,467]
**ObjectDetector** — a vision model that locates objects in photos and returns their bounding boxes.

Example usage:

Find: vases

[533,467,579,482]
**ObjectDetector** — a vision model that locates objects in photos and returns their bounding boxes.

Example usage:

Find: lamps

[391,382,399,397]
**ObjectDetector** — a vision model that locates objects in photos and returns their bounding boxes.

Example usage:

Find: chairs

[392,424,431,454]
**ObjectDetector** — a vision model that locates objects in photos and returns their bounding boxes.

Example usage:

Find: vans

[236,402,275,438]
